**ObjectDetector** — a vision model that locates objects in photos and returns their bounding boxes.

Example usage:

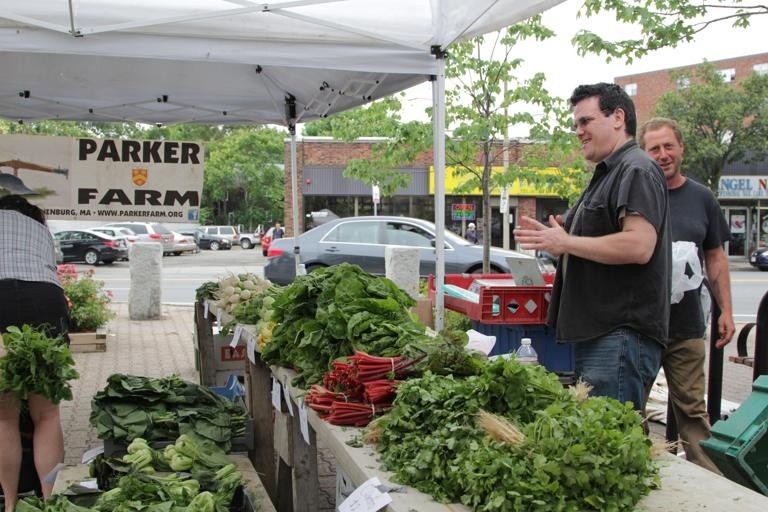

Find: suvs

[103,219,174,257]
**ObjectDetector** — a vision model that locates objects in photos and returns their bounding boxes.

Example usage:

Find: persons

[639,117,735,476]
[512,82,671,430]
[464,223,478,244]
[0,194,72,512]
[272,222,284,240]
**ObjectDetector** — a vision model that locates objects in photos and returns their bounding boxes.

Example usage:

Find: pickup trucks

[232,223,266,250]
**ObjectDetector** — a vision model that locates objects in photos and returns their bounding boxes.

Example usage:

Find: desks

[205,289,768,511]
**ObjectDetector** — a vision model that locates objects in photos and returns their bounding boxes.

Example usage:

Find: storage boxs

[212,328,247,369]
[215,367,252,415]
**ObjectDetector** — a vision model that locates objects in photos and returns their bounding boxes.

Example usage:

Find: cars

[194,227,233,251]
[167,230,196,257]
[261,224,285,256]
[748,246,768,271]
[261,214,555,289]
[50,229,128,266]
[87,227,143,259]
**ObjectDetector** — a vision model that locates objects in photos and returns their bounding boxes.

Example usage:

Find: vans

[197,225,240,246]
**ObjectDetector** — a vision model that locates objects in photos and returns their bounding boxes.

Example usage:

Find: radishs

[212,271,272,313]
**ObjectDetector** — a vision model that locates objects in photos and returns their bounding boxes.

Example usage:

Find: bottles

[295,264,306,277]
[516,338,538,366]
